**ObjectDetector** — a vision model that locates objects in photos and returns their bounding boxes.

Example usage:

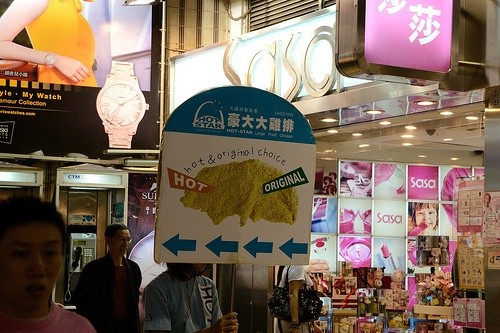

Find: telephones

[71,245,94,272]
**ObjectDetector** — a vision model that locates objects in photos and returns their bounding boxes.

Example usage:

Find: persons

[0,0,100,87]
[273,265,313,333]
[412,202,450,267]
[142,263,239,333]
[483,192,494,213]
[69,223,141,333]
[0,197,98,333]
[451,248,485,333]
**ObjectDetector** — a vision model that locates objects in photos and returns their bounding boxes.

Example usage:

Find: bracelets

[289,323,299,329]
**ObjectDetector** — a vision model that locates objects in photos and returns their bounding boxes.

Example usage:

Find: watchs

[128,230,168,325]
[95,61,149,149]
[46,52,56,68]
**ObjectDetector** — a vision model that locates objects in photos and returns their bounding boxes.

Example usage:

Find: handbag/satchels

[268,265,323,322]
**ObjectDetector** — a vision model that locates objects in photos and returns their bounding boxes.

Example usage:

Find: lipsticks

[316,242,327,261]
[380,243,397,275]
